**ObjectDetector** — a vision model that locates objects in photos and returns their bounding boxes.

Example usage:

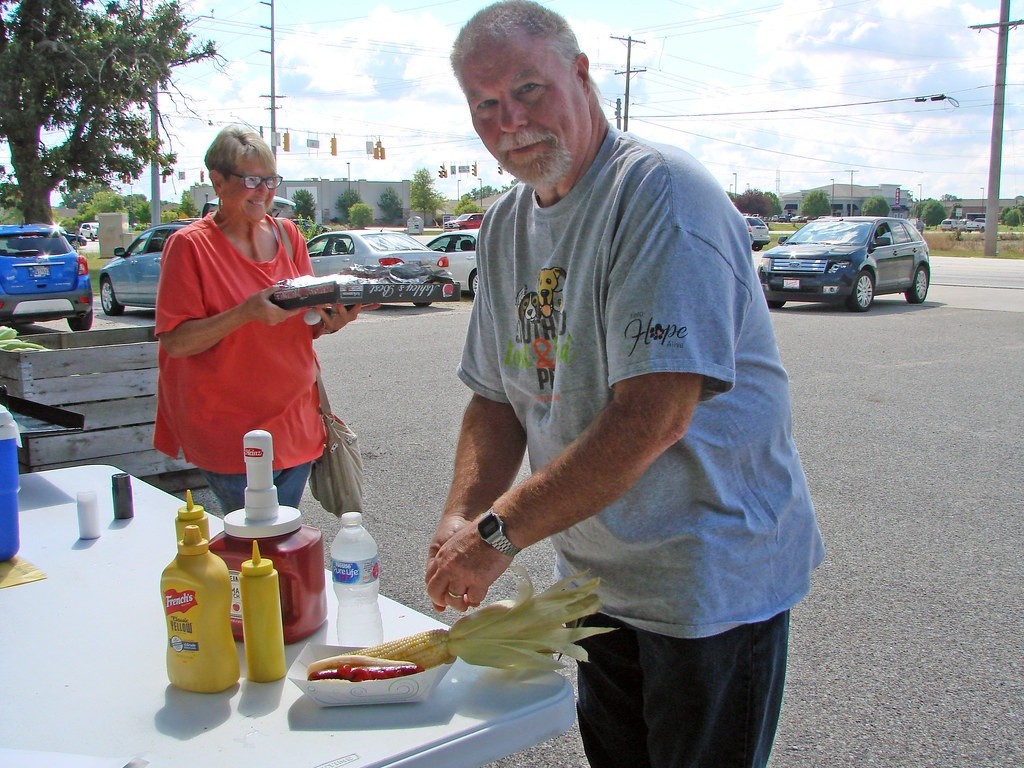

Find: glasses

[215,166,283,189]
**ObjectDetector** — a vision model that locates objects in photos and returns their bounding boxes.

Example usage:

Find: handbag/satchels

[306,414,362,513]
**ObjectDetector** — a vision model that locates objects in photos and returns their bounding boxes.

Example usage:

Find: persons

[426,0,824,768]
[153,124,380,516]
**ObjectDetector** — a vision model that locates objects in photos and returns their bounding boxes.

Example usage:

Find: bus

[965,213,986,224]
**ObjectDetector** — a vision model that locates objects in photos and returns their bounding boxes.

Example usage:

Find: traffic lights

[498,163,503,174]
[438,166,447,178]
[201,171,204,182]
[472,164,477,176]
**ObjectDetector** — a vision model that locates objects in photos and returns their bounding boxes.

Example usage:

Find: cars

[100,219,197,315]
[751,214,822,223]
[59,229,88,247]
[306,230,483,307]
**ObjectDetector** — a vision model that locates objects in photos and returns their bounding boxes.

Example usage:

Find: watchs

[477,506,522,558]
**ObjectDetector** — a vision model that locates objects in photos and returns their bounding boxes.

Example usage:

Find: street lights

[458,178,482,206]
[347,163,351,190]
[730,172,737,197]
[831,179,834,206]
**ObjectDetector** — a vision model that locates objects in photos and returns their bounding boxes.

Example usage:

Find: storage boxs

[270,281,460,310]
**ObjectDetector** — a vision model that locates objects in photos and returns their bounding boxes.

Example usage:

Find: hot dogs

[308,654,424,681]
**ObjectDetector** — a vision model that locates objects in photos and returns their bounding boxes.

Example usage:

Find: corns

[0,326,48,350]
[339,628,452,671]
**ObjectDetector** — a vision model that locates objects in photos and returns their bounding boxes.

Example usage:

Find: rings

[447,589,465,599]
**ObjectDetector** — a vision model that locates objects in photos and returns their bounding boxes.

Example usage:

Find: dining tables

[0,465,575,768]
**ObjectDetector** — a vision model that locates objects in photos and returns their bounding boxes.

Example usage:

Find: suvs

[942,219,961,230]
[966,218,986,232]
[0,223,93,332]
[445,213,485,229]
[757,216,931,312]
[744,215,770,252]
[81,222,100,241]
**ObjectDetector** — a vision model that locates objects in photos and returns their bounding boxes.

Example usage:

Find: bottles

[111,472,134,520]
[203,504,330,645]
[76,490,101,540]
[159,489,289,694]
[330,512,384,649]
